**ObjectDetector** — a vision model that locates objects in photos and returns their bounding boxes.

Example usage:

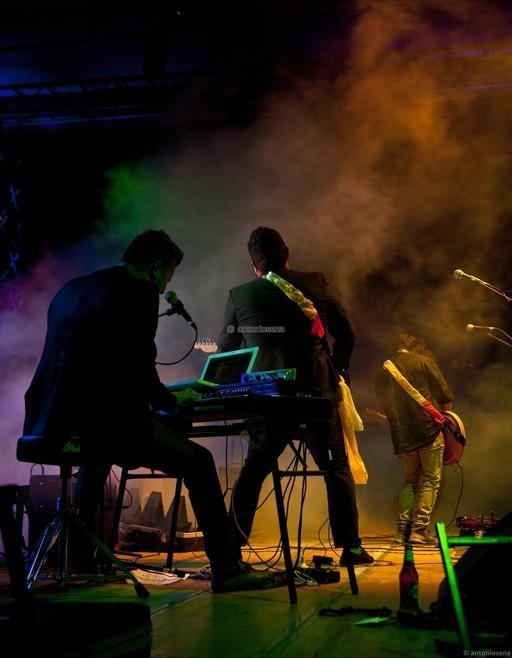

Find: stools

[16,435,152,600]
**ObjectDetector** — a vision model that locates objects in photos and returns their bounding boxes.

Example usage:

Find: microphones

[164,290,197,330]
[466,324,495,333]
[453,269,489,286]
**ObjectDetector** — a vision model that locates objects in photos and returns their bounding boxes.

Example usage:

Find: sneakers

[395,530,438,545]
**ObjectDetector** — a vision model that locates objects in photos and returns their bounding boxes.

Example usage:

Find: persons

[379,330,454,546]
[21,227,277,595]
[193,224,377,566]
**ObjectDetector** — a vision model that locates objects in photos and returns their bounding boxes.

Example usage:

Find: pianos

[170,380,333,426]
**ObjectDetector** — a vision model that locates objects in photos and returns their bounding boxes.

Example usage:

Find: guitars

[193,336,351,387]
[364,406,465,464]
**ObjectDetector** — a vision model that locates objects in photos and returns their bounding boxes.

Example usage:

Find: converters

[313,556,333,572]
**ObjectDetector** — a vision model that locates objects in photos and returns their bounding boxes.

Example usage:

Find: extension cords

[292,567,340,584]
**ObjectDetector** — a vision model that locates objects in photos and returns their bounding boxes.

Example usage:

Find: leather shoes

[340,546,374,565]
[211,560,274,592]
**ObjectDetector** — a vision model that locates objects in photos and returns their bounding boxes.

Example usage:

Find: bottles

[399,545,419,612]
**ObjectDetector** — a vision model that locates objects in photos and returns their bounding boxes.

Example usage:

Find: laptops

[163,346,259,391]
